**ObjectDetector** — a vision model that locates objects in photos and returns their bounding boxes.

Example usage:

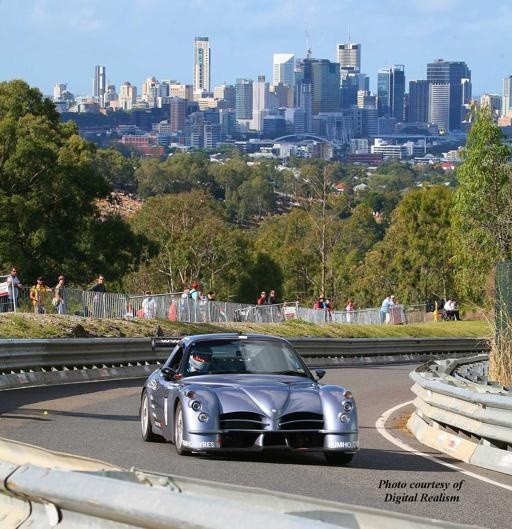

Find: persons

[6,265,461,326]
[187,347,213,373]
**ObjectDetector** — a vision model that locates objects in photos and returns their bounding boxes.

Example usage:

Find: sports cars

[136,331,362,466]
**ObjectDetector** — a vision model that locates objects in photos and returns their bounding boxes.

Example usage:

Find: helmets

[189,347,213,371]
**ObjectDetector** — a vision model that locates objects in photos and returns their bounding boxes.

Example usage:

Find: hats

[59,276,65,280]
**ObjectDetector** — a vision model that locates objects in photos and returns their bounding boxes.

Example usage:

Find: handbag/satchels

[188,291,197,298]
[52,298,60,307]
